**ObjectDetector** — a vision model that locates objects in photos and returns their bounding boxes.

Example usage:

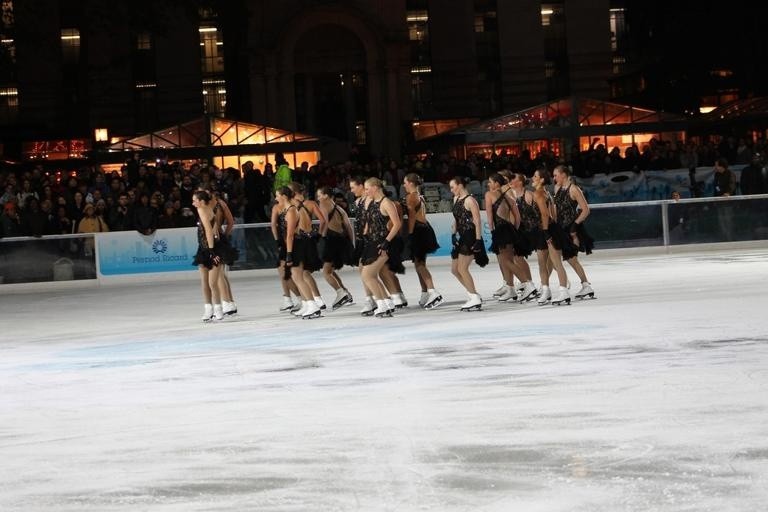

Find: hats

[4,203,15,209]
[82,204,96,213]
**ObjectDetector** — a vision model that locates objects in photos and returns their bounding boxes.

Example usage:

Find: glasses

[25,176,32,178]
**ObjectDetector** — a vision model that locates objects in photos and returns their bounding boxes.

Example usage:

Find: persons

[2,137,767,280]
[192,164,596,323]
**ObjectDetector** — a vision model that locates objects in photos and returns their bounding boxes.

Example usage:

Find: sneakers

[291,296,302,311]
[423,289,442,308]
[537,288,552,298]
[575,282,594,297]
[202,304,213,320]
[551,286,570,302]
[332,288,352,308]
[538,285,551,303]
[360,294,378,314]
[390,293,407,306]
[374,300,390,315]
[302,300,320,316]
[419,291,428,305]
[280,294,293,309]
[384,298,395,309]
[314,296,327,309]
[213,301,237,317]
[461,293,482,308]
[293,300,308,316]
[517,281,537,302]
[214,304,224,320]
[494,281,507,296]
[499,285,517,300]
